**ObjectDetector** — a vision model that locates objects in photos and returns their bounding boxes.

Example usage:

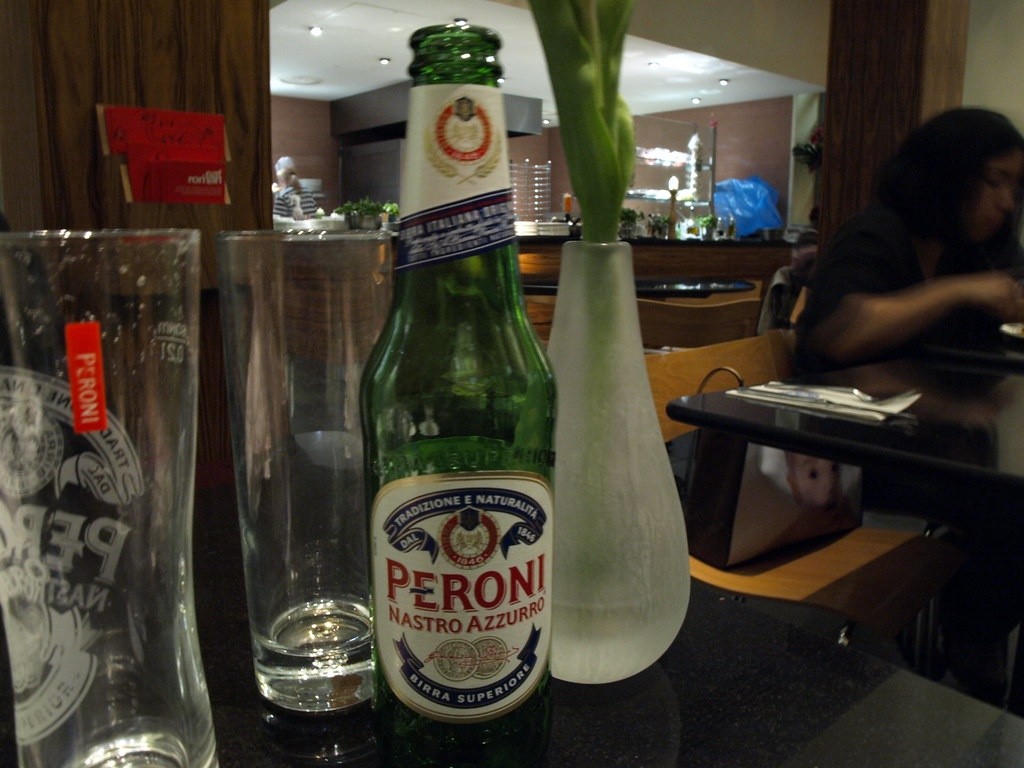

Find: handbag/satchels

[665,366,866,569]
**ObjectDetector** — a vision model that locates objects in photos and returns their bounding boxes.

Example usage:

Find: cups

[1,227,218,768]
[222,227,397,713]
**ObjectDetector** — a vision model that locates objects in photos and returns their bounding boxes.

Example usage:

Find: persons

[271,156,318,219]
[792,107,1024,711]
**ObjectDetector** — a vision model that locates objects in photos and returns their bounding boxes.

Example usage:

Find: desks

[1,576,1023,768]
[664,354,1024,657]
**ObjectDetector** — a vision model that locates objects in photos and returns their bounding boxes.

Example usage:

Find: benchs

[641,333,942,635]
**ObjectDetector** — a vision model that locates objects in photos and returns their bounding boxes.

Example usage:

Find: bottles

[727,218,736,240]
[355,18,559,721]
[687,207,701,239]
[717,217,724,240]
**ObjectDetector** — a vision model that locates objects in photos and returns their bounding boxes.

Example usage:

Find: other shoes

[943,627,1007,703]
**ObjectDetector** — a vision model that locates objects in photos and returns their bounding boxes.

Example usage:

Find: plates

[513,220,570,237]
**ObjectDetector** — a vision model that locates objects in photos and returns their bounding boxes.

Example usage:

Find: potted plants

[617,209,643,240]
[701,213,719,239]
[648,216,672,239]
[726,220,736,238]
[338,197,401,232]
